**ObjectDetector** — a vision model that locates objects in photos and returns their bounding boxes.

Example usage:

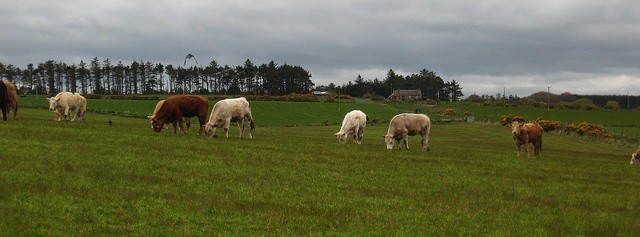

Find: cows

[151,94,209,135]
[4,81,18,118]
[0,80,11,121]
[381,113,432,150]
[629,147,639,165]
[334,109,368,145]
[46,91,87,122]
[148,100,192,130]
[201,97,256,140]
[507,120,544,159]
